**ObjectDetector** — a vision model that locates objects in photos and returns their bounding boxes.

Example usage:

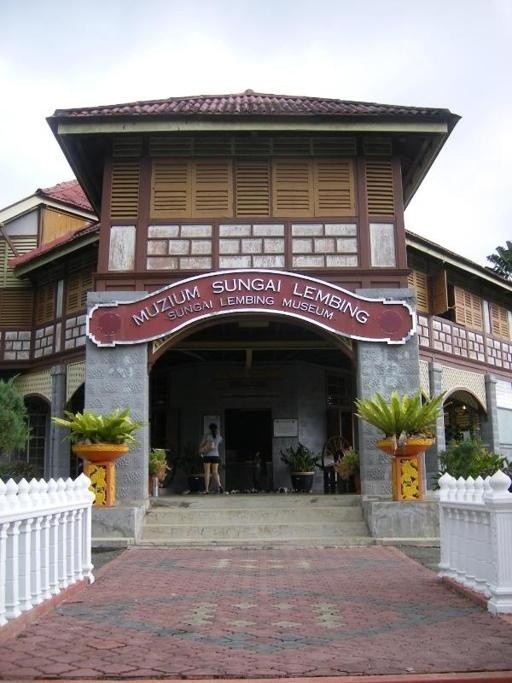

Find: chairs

[322,435,353,494]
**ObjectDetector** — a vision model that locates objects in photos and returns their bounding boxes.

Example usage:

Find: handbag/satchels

[198,440,214,453]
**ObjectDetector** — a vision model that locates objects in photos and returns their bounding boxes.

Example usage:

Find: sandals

[198,489,209,494]
[217,486,223,495]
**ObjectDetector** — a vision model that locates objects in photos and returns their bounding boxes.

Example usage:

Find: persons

[323,442,356,492]
[439,304,457,320]
[445,424,468,445]
[224,450,268,495]
[200,423,223,495]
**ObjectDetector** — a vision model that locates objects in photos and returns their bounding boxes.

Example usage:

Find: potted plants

[280,444,321,492]
[48,409,146,508]
[338,447,360,494]
[353,387,453,502]
[149,447,172,493]
[173,441,212,493]
